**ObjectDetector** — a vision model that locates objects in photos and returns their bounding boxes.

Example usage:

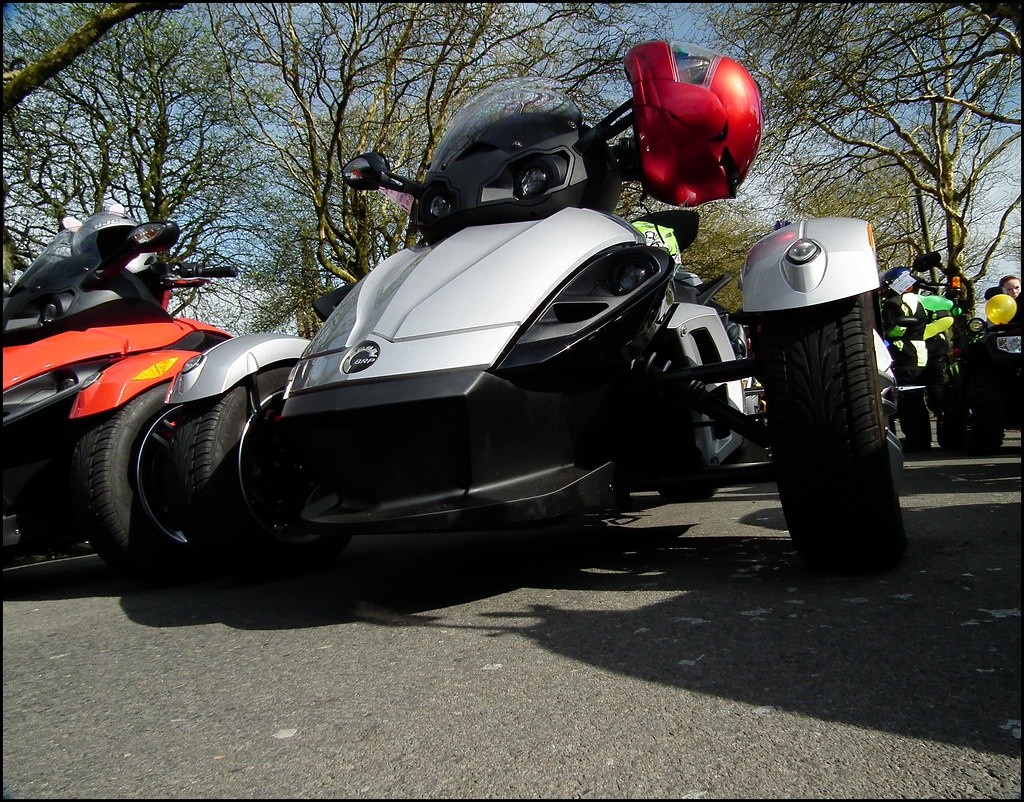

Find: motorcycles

[0,201,236,591]
[160,38,911,592]
[936,322,1023,455]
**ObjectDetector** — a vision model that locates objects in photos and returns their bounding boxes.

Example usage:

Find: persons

[987,275,1024,326]
[879,266,949,421]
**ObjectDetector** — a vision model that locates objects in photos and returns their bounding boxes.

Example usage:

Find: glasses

[1003,275,1018,280]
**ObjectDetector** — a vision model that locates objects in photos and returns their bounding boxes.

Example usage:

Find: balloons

[985,294,1017,325]
[923,317,954,340]
[915,293,954,311]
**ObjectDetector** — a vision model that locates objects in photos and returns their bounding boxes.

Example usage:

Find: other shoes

[899,438,931,451]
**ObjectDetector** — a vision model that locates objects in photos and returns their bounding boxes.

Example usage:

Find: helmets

[74,211,170,311]
[879,265,920,297]
[624,40,763,208]
